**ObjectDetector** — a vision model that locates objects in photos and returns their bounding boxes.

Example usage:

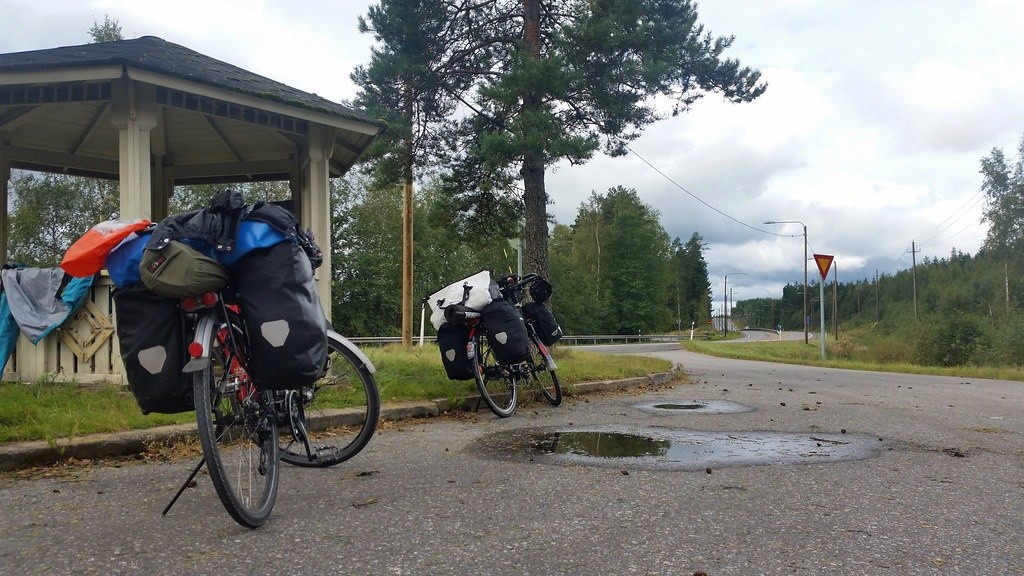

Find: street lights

[764,221,808,344]
[725,273,746,337]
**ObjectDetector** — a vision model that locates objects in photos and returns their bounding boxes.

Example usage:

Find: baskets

[500,283,524,305]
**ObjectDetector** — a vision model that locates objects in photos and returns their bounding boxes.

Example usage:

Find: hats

[530,276,553,304]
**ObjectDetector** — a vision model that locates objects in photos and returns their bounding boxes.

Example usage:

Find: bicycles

[162,266,380,529]
[466,273,561,418]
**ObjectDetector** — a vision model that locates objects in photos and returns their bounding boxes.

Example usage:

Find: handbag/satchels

[421,270,502,331]
[521,301,563,346]
[60,190,323,297]
[236,240,329,388]
[481,297,530,364]
[296,227,323,270]
[110,284,220,415]
[437,323,482,380]
[497,274,521,291]
